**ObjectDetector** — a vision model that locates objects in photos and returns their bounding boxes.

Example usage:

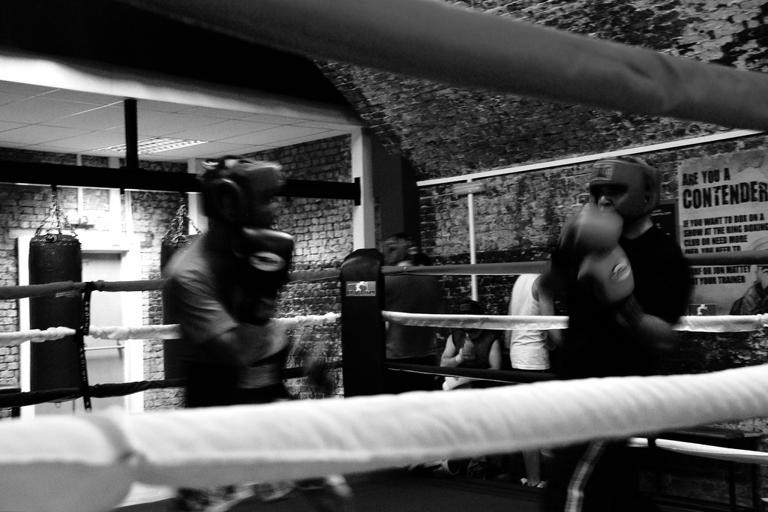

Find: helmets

[589,154,660,221]
[200,157,285,227]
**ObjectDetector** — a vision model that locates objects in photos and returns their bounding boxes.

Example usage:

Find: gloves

[555,202,639,304]
[227,224,294,322]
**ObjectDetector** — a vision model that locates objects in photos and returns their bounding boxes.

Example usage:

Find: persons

[377,232,446,473]
[696,234,768,433]
[535,156,699,511]
[440,302,508,481]
[161,156,352,511]
[505,271,565,495]
